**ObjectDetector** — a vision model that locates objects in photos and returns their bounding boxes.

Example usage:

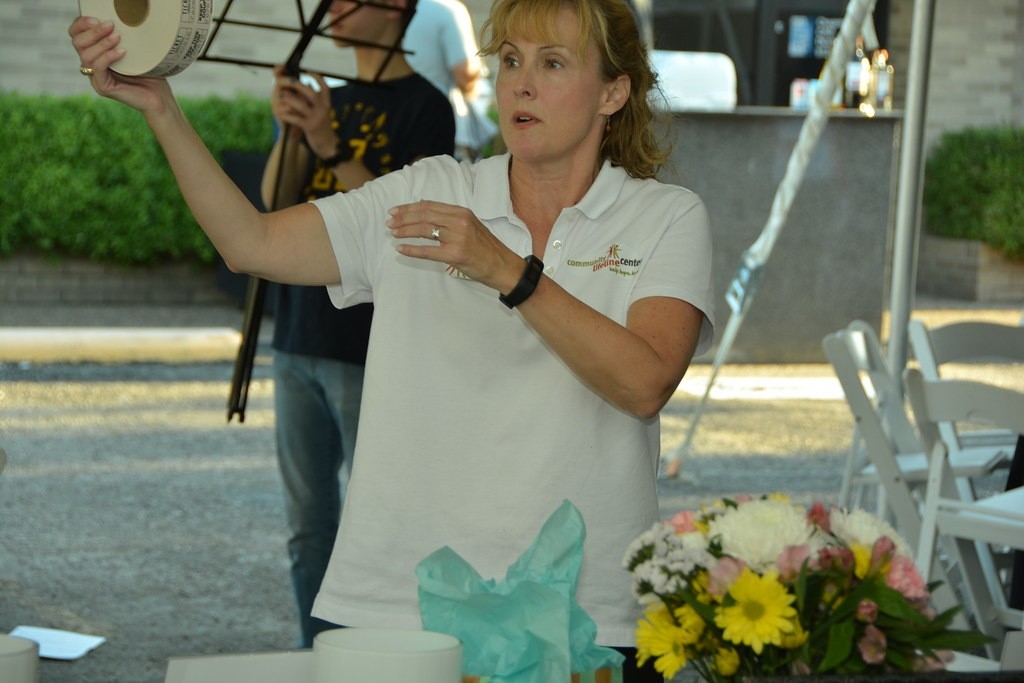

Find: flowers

[621,496,991,683]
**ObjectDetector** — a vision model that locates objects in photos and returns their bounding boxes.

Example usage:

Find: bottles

[789,509,855,670]
[843,37,871,109]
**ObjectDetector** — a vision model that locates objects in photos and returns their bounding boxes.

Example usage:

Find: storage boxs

[462,668,612,683]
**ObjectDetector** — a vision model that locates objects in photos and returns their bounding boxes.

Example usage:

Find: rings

[431,224,441,242]
[79,63,94,78]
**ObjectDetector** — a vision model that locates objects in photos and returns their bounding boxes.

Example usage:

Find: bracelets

[498,253,545,310]
[319,137,357,171]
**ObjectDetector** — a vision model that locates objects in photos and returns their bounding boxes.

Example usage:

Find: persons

[67,1,718,683]
[260,2,500,652]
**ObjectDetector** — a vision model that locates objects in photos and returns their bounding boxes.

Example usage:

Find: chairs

[902,367,1024,660]
[909,320,1019,609]
[824,319,1024,638]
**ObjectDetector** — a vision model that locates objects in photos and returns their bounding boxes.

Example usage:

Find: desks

[632,108,908,365]
[163,650,313,683]
